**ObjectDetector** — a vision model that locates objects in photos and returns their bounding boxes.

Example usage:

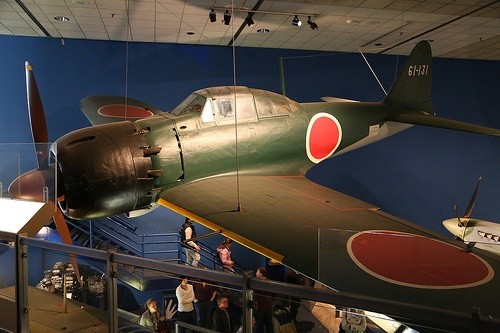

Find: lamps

[307,16,319,30]
[244,12,255,26]
[209,9,217,22]
[292,15,302,26]
[222,10,231,26]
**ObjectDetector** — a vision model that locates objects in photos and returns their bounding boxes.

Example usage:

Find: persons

[176,277,199,333]
[180,218,201,267]
[217,239,235,273]
[212,295,234,333]
[139,298,164,333]
[341,307,367,333]
[254,257,311,333]
[192,265,217,328]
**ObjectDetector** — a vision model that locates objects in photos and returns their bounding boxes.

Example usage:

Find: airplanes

[7,39,500,333]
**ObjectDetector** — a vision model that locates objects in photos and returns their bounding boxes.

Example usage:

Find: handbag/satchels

[338,308,347,333]
